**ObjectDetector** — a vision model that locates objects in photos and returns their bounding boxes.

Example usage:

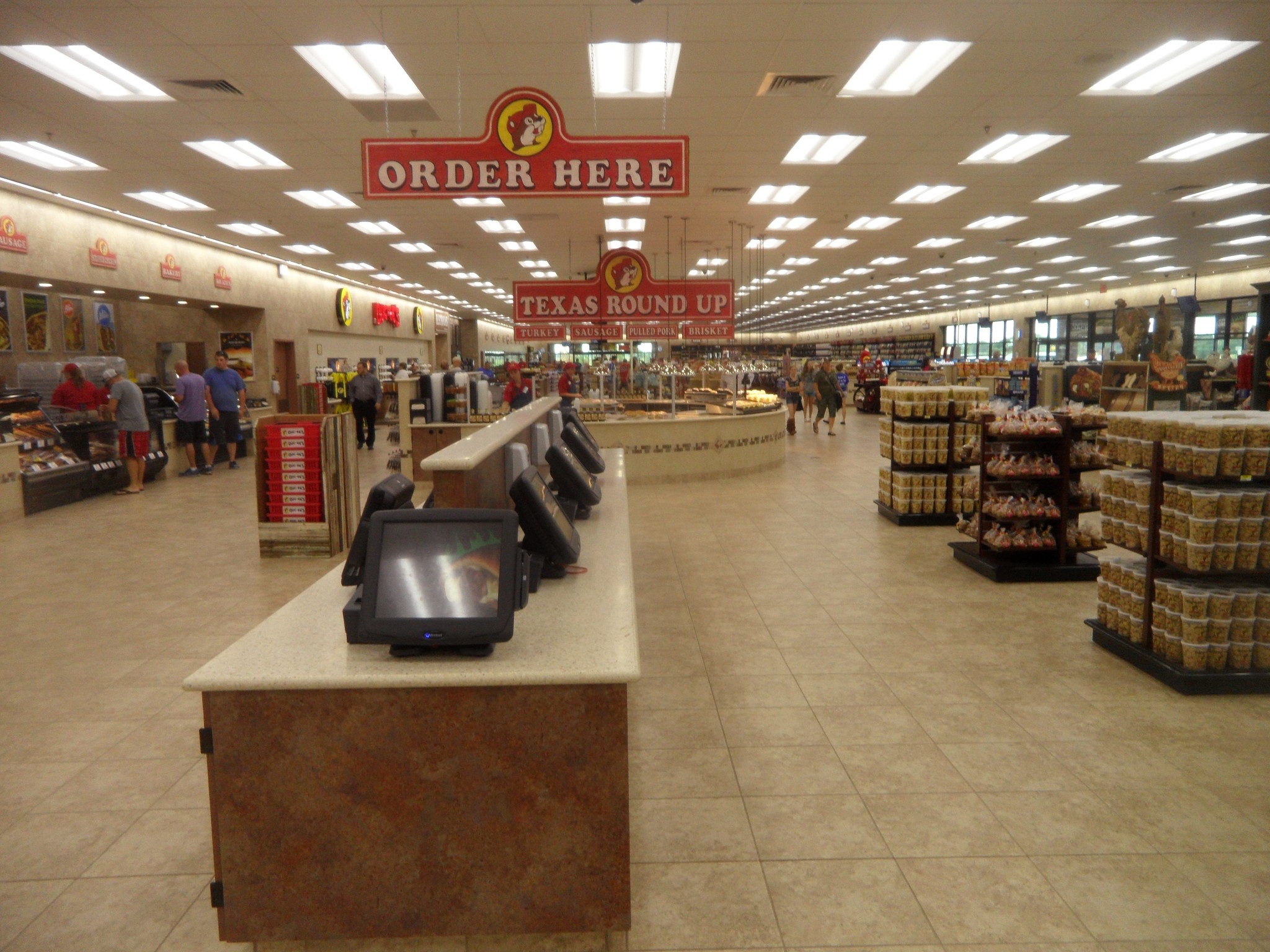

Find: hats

[60,363,78,372]
[822,356,832,362]
[101,369,117,381]
[564,362,577,369]
[507,362,520,372]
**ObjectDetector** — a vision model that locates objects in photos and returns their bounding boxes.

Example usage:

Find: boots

[786,417,797,434]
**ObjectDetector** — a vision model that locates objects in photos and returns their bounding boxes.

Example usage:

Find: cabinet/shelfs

[410,427,462,483]
[873,411,983,528]
[252,412,360,562]
[948,414,1108,587]
[1081,457,1270,693]
[1097,358,1153,412]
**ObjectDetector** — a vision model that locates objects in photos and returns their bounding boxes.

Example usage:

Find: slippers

[115,488,144,493]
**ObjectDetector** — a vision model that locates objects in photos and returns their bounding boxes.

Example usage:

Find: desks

[181,444,636,952]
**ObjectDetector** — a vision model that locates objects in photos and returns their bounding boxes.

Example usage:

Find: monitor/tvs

[1176,295,1201,314]
[341,473,416,586]
[979,317,991,328]
[359,508,518,643]
[508,407,606,564]
[1035,310,1048,323]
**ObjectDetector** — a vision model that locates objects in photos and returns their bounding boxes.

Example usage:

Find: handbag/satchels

[835,392,842,410]
[795,396,804,412]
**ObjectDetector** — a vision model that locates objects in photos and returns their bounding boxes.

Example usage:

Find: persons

[451,356,463,370]
[1081,347,1099,361]
[440,359,451,371]
[348,361,384,451]
[50,361,99,418]
[173,359,212,475]
[99,369,152,496]
[800,359,815,422]
[784,365,800,435]
[988,348,1004,361]
[557,362,582,407]
[823,364,849,425]
[812,358,845,436]
[203,349,248,469]
[477,362,495,380]
[501,361,539,413]
[392,362,409,380]
[918,352,931,372]
[504,355,661,393]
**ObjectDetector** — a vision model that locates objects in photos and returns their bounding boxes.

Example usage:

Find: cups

[877,385,989,514]
[1095,407,1269,671]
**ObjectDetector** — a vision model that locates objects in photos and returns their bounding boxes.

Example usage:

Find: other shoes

[357,439,366,449]
[179,468,201,476]
[828,432,836,435]
[804,418,811,423]
[229,460,239,468]
[823,419,829,424]
[813,421,818,433]
[368,446,373,449]
[203,464,214,468]
[203,466,212,475]
[840,421,846,424]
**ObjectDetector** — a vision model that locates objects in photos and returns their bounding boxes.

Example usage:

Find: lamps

[976,304,993,328]
[1176,272,1202,313]
[1036,296,1052,323]
